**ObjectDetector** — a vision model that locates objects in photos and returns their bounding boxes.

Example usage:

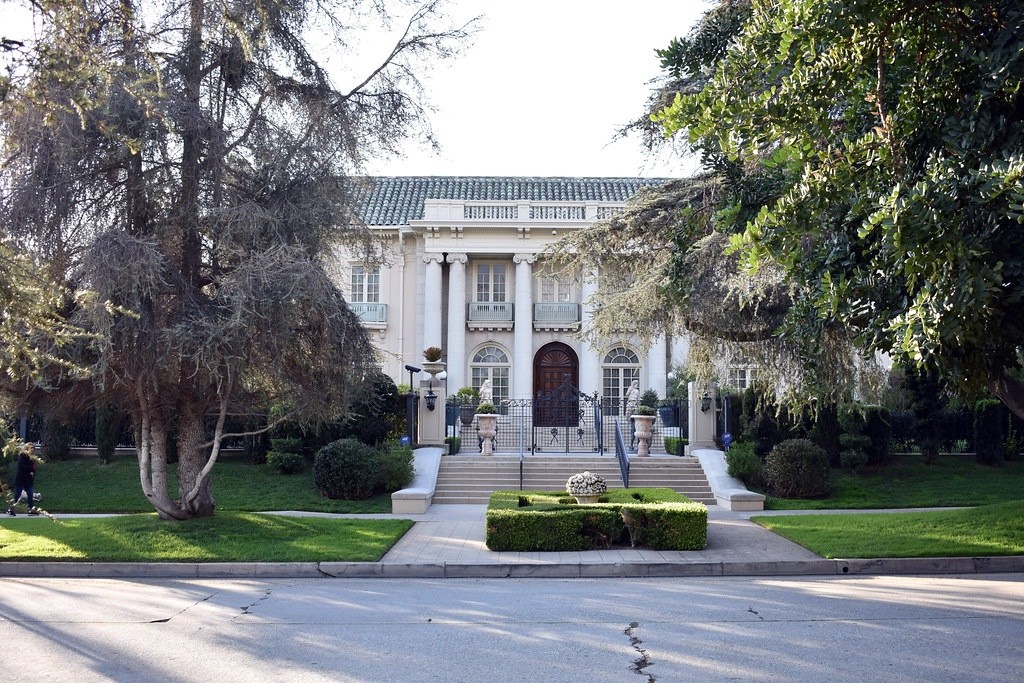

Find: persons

[626,380,639,415]
[6,442,40,516]
[478,380,492,404]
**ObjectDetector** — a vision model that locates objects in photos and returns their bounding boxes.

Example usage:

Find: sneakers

[27,510,39,516]
[6,509,16,517]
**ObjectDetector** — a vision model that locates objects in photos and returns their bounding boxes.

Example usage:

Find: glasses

[29,447,35,450]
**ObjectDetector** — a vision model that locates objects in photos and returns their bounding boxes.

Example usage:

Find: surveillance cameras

[551,230,557,235]
[405,365,421,372]
